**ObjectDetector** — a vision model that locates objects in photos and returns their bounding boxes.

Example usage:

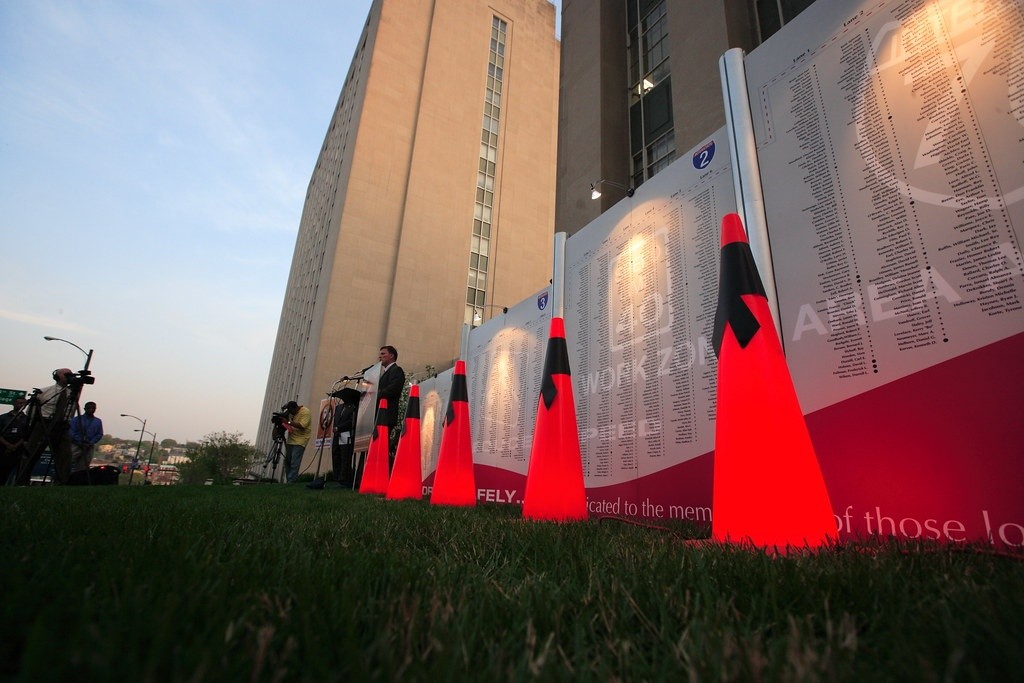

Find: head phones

[53,369,60,382]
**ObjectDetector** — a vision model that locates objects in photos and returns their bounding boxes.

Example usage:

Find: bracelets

[288,421,291,424]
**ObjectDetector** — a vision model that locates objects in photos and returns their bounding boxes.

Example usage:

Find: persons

[0,398,28,486]
[374,345,405,455]
[279,401,312,483]
[21,368,75,487]
[70,402,103,472]
[331,403,354,482]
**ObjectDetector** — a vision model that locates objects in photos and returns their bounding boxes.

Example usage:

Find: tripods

[256,429,290,489]
[0,385,92,487]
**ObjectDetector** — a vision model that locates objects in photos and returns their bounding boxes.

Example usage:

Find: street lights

[134,428,157,483]
[43,334,94,370]
[120,412,147,485]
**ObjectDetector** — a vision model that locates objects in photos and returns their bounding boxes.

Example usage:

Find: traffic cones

[430,360,478,509]
[521,318,590,526]
[385,384,424,501]
[358,394,391,496]
[683,212,847,562]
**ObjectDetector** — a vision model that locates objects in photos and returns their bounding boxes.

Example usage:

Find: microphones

[358,364,374,373]
